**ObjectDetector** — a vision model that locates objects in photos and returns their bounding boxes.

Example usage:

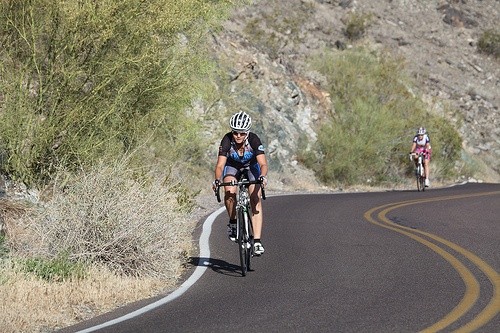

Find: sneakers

[253,242,264,255]
[227,223,237,241]
[425,179,429,187]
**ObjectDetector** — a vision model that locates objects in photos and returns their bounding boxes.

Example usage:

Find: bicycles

[406,148,432,192]
[211,174,269,278]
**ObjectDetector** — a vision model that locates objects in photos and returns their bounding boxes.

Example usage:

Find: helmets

[417,127,426,134]
[229,111,252,133]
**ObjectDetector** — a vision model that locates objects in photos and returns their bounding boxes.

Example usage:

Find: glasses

[418,134,423,136]
[232,131,246,136]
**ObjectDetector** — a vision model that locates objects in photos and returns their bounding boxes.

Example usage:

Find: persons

[409,126,431,187]
[213,110,268,256]
